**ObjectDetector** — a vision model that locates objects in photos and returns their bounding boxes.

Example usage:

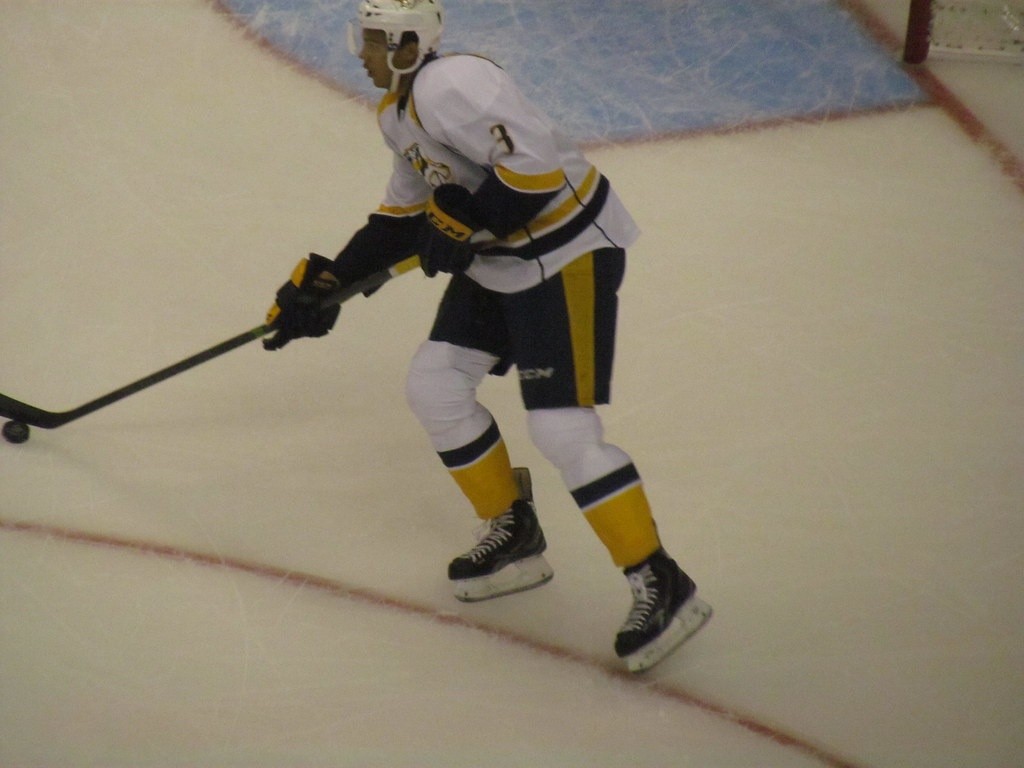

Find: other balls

[2,421,29,443]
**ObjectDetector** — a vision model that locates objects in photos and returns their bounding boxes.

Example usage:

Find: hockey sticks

[0,254,422,429]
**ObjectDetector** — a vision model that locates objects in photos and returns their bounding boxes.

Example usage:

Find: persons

[258,1,713,673]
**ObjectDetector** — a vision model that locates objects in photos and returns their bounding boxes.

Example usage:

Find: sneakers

[446,501,555,601]
[615,546,713,673]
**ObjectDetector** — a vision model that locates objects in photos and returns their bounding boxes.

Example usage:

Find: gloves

[416,186,477,275]
[264,253,344,352]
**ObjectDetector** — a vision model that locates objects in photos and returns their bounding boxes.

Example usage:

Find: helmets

[347,0,442,92]
